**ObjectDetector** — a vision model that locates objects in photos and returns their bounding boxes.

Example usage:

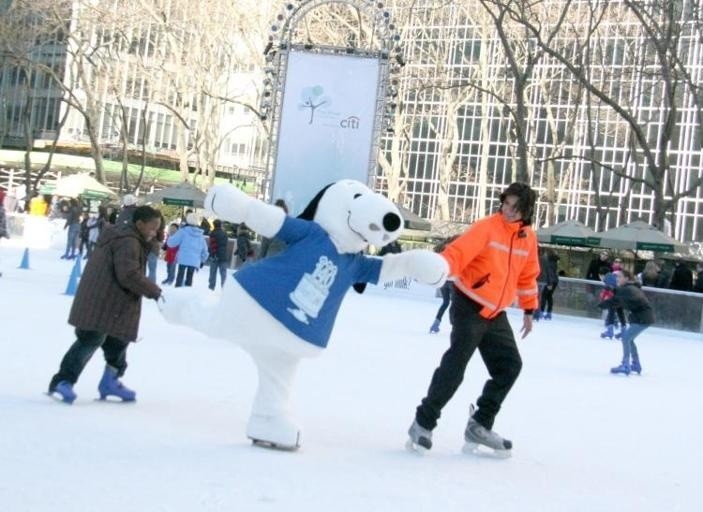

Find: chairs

[465,402,515,451]
[407,417,435,449]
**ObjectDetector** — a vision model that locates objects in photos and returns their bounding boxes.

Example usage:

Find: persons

[407,182,540,448]
[670,258,693,291]
[600,257,627,339]
[533,248,558,320]
[381,241,400,256]
[586,250,612,281]
[431,234,462,333]
[48,206,166,403]
[2,187,20,240]
[28,194,48,217]
[60,199,289,290]
[596,268,656,376]
[642,261,659,287]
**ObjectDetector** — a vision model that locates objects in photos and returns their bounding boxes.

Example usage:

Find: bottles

[184,212,205,228]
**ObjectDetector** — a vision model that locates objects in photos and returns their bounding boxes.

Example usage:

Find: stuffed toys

[157,178,449,449]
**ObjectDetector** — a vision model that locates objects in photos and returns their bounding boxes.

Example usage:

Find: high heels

[74,256,82,277]
[17,248,32,269]
[60,266,81,296]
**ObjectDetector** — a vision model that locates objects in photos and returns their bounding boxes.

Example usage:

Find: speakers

[50,378,77,401]
[537,312,552,319]
[609,362,632,375]
[614,326,627,339]
[600,325,614,337]
[97,364,137,402]
[429,318,441,333]
[630,360,642,373]
